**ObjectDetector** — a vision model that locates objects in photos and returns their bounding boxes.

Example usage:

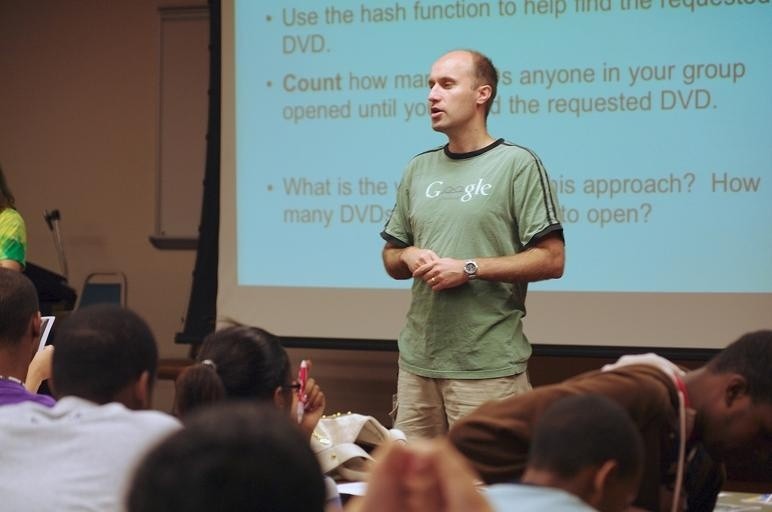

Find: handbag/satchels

[309,412,408,496]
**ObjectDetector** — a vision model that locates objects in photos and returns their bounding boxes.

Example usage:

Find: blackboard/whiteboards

[152,5,209,249]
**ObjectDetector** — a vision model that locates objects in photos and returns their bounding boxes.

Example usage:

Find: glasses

[287,378,301,393]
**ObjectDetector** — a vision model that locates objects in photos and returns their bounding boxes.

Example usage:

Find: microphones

[41,208,68,281]
[52,207,68,280]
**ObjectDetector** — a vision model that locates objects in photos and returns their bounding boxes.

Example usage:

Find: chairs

[71,270,128,316]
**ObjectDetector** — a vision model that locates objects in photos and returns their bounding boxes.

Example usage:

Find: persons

[1,267,772,510]
[379,50,567,447]
[1,166,28,272]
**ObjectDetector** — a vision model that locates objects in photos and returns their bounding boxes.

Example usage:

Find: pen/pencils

[297,360,307,424]
